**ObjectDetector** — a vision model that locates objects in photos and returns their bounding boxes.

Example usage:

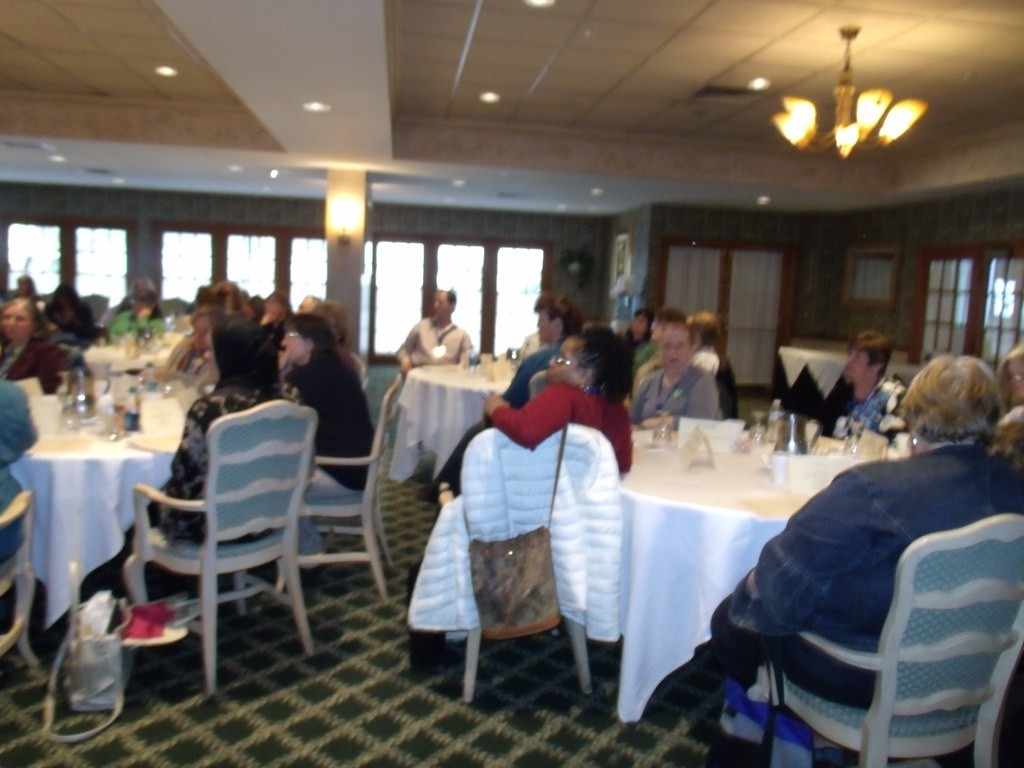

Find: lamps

[771,24,930,156]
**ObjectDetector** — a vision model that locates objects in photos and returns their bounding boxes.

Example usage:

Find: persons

[0,278,374,669]
[397,289,475,379]
[434,295,1024,767]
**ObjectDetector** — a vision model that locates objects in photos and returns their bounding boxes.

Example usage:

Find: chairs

[0,295,1024,768]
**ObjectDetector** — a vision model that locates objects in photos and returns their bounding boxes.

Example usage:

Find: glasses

[549,355,573,367]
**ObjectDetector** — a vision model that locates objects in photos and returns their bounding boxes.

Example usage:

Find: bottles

[773,413,807,454]
[122,387,140,437]
[767,399,785,444]
[467,346,477,374]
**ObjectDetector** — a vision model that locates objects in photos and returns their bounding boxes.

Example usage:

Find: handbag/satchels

[60,563,134,712]
[468,526,561,639]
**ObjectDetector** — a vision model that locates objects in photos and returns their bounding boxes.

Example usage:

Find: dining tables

[12,388,181,630]
[780,346,852,398]
[83,340,166,377]
[603,414,923,724]
[390,363,520,483]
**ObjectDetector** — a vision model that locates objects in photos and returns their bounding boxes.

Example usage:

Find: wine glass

[652,409,672,446]
[57,367,177,416]
[843,420,865,456]
[749,410,766,443]
